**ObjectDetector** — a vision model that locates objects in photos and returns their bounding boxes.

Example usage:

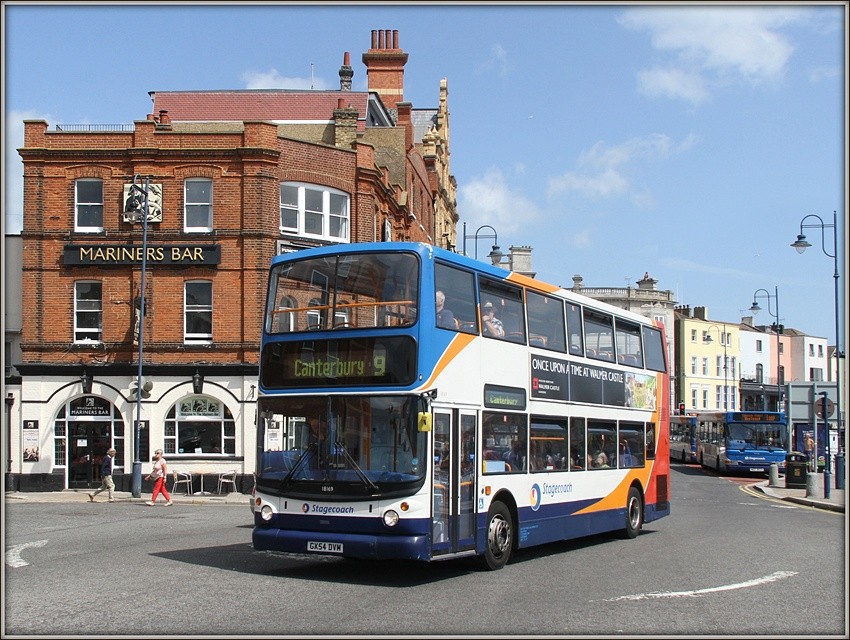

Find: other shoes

[109,501,117,504]
[145,501,155,506]
[164,501,173,507]
[89,494,94,503]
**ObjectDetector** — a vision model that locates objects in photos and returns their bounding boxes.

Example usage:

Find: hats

[482,302,497,312]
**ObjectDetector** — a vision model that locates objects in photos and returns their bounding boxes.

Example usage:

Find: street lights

[128,171,157,498]
[790,211,844,491]
[751,287,783,409]
[462,219,500,261]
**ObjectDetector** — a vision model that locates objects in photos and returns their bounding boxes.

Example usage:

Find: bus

[693,410,789,473]
[669,413,692,463]
[249,239,669,573]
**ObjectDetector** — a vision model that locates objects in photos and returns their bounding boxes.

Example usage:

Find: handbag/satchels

[150,471,160,481]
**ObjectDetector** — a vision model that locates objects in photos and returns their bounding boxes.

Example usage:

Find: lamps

[192,369,203,392]
[80,371,92,393]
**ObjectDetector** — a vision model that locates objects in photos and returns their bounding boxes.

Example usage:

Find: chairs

[217,470,238,495]
[171,469,193,495]
[454,319,545,346]
[570,344,639,365]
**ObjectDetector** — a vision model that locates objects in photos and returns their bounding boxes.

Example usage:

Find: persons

[458,300,504,339]
[303,413,358,470]
[647,428,654,448]
[145,449,173,507]
[439,439,632,484]
[804,433,815,472]
[88,448,115,503]
[436,290,457,330]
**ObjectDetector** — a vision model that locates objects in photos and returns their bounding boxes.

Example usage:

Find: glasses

[155,452,160,454]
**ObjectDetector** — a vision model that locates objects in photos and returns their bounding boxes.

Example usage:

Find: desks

[191,470,215,495]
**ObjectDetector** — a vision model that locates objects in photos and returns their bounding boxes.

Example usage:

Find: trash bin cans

[785,452,808,489]
